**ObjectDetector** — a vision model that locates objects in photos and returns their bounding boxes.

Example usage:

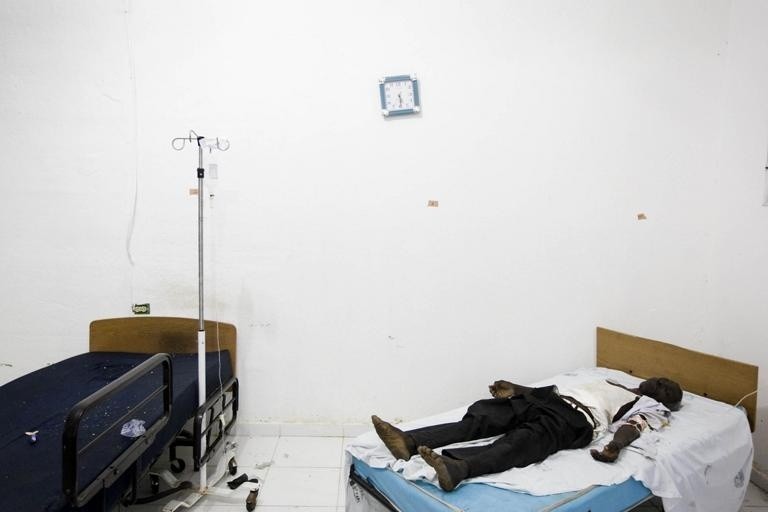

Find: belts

[561,395,595,429]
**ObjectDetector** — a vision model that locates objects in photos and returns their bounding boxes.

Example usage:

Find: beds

[0,311,240,512]
[347,325,758,512]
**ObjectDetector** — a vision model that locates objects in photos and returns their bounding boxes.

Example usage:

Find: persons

[372,369,684,494]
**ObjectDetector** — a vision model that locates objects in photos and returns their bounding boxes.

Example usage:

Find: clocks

[377,73,423,120]
